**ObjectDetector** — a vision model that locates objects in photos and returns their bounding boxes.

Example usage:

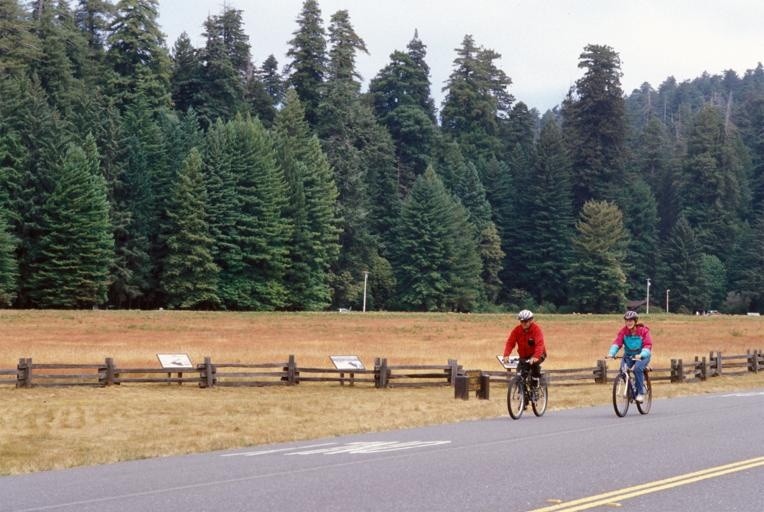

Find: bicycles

[502,358,548,419]
[605,355,652,417]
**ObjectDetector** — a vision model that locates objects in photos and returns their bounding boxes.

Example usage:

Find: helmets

[517,310,533,321]
[623,311,638,320]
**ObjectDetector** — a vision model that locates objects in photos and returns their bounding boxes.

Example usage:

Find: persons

[606,310,653,404]
[503,309,547,411]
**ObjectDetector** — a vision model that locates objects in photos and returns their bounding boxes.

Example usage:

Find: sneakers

[519,391,536,410]
[622,389,643,403]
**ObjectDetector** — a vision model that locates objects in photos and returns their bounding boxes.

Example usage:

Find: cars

[708,311,718,314]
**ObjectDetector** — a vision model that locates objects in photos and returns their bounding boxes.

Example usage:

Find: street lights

[667,289,671,312]
[646,278,651,314]
[362,271,370,312]
